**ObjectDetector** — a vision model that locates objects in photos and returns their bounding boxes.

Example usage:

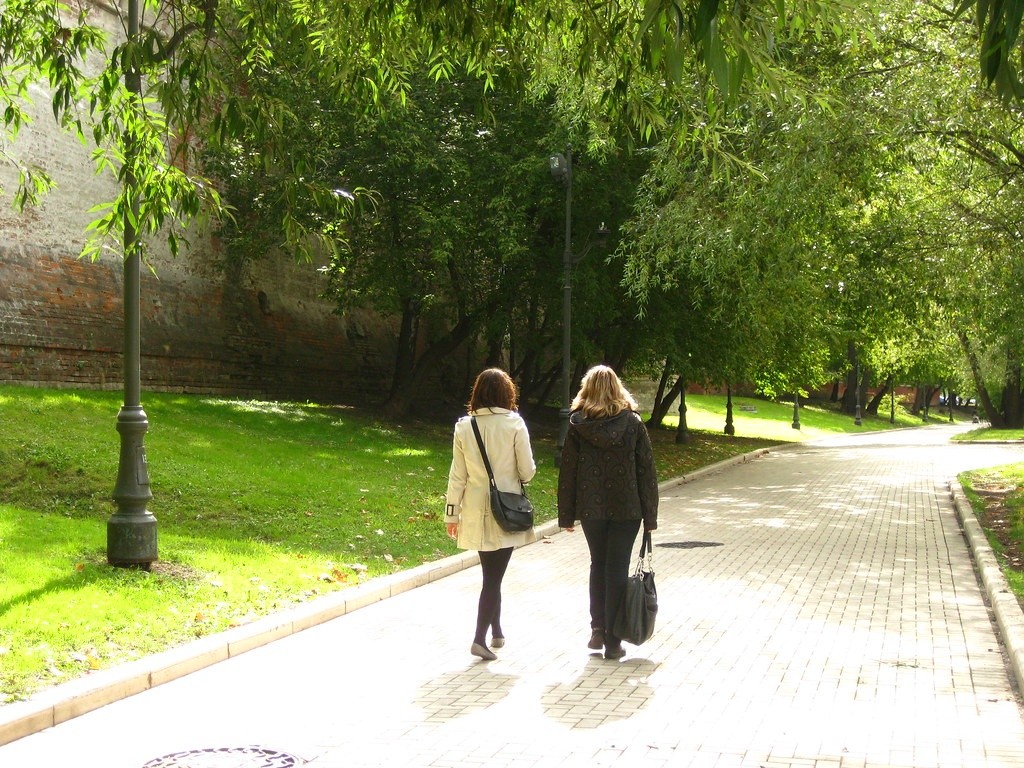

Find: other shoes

[589,631,626,659]
[470,637,504,660]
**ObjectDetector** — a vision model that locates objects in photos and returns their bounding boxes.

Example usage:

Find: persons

[444,368,536,661]
[557,366,660,660]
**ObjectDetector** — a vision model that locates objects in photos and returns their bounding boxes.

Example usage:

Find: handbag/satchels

[612,532,659,646]
[492,488,534,531]
